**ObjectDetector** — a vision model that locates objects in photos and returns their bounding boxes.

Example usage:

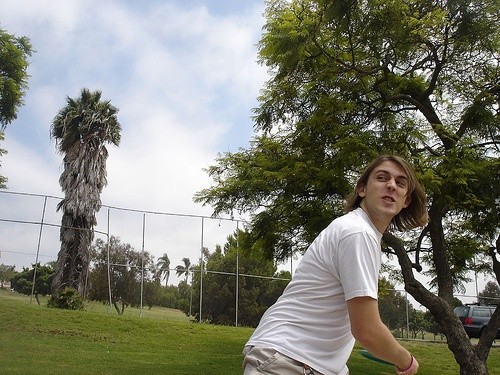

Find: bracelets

[396,353,415,374]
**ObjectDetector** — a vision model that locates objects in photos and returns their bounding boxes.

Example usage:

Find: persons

[240,154,429,375]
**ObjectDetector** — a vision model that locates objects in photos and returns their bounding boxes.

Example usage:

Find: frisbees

[359,349,394,366]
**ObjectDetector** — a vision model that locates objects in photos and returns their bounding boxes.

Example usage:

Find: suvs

[453,305,500,340]
